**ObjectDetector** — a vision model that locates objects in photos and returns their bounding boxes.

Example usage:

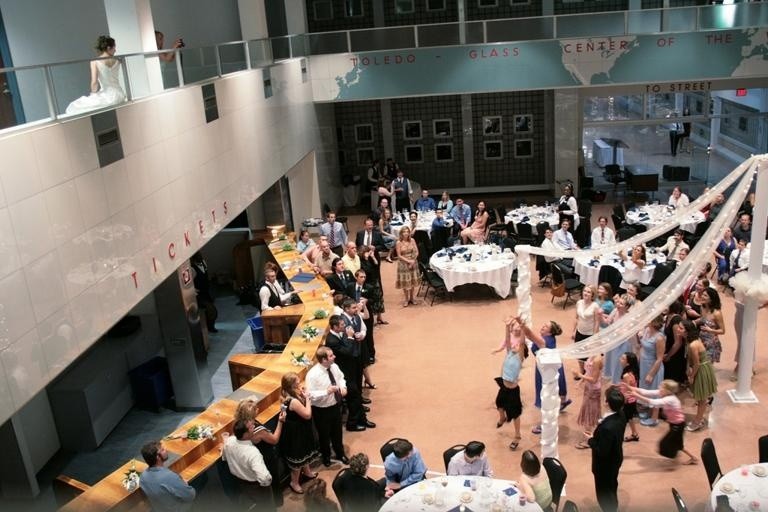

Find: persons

[220,400,286,511]
[515,449,553,510]
[417,191,489,244]
[343,453,381,511]
[666,111,683,157]
[259,263,303,312]
[447,441,493,477]
[304,478,339,512]
[140,441,199,512]
[396,212,419,306]
[572,229,726,511]
[493,313,572,449]
[541,216,615,279]
[679,107,692,155]
[279,347,347,493]
[558,184,580,235]
[154,30,185,87]
[66,36,127,114]
[192,250,218,333]
[367,157,413,262]
[668,184,756,292]
[384,439,427,498]
[296,213,387,432]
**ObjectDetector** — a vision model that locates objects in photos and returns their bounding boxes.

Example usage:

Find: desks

[593,140,623,168]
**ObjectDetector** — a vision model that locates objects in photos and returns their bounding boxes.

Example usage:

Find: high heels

[377,317,388,324]
[686,418,706,432]
[364,380,376,389]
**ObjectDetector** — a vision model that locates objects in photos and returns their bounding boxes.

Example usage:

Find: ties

[601,229,604,243]
[327,368,342,407]
[330,226,335,250]
[340,274,348,289]
[352,318,357,326]
[459,206,465,225]
[399,177,402,183]
[355,286,363,304]
[367,232,368,246]
[564,232,571,249]
[666,245,678,261]
[731,251,743,275]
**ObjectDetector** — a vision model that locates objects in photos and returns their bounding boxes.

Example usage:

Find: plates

[717,464,766,512]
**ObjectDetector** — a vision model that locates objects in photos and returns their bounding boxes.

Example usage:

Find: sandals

[624,434,639,441]
[510,436,521,450]
[496,417,506,428]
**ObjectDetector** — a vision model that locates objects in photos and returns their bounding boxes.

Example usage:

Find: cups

[436,240,513,269]
[390,208,447,229]
[627,200,678,222]
[384,475,527,512]
[514,200,559,223]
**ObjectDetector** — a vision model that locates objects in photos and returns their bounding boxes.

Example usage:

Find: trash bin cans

[247,317,264,351]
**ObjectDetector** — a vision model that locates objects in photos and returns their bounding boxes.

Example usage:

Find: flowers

[270,234,286,243]
[300,323,320,343]
[163,423,214,441]
[121,455,140,492]
[288,349,312,367]
[302,308,330,324]
[274,243,292,255]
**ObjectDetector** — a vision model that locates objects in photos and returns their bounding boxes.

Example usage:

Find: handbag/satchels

[550,281,565,297]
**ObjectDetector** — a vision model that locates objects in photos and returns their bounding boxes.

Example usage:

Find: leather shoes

[348,398,375,431]
[323,454,330,466]
[339,455,349,466]
[684,459,698,466]
[289,482,304,494]
[385,257,393,263]
[532,426,540,433]
[303,471,318,481]
[404,299,417,307]
[560,398,570,410]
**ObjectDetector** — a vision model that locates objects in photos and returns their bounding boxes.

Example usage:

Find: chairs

[671,435,768,512]
[332,437,579,512]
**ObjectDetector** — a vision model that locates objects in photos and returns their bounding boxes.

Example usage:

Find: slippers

[575,441,590,449]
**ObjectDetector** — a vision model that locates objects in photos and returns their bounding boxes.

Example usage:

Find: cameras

[178,39,185,47]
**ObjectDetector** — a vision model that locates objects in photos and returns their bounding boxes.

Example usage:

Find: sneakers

[639,411,659,425]
